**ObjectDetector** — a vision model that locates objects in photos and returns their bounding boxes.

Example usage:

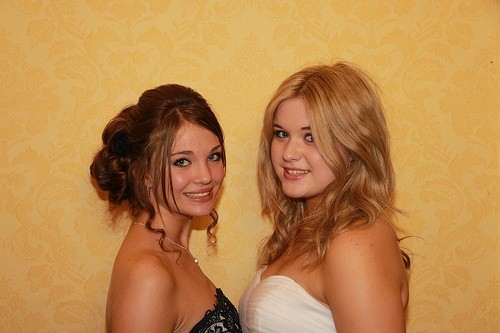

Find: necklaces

[130,223,198,263]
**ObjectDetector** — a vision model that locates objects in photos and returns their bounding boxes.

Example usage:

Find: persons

[238,60,408,332]
[89,84,242,333]
